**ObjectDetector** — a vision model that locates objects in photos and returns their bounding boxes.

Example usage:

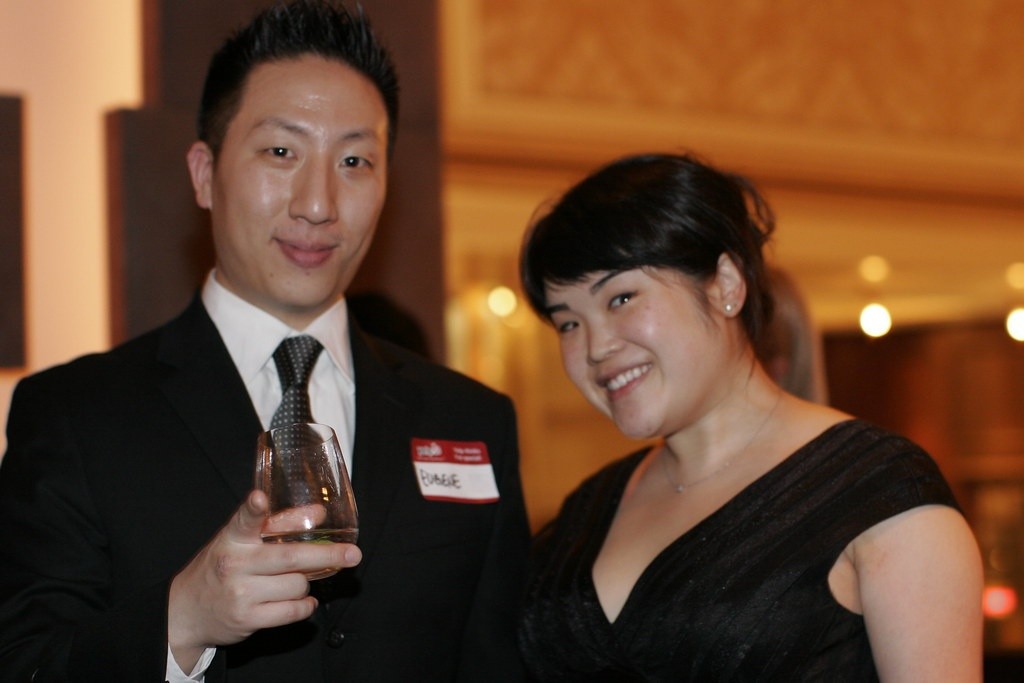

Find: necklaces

[658,395,781,493]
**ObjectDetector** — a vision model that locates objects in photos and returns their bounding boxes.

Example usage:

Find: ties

[268,335,339,537]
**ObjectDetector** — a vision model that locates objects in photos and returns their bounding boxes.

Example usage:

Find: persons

[0,1,533,683]
[507,151,986,682]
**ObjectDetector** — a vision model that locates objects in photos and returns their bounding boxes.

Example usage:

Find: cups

[254,424,362,582]
